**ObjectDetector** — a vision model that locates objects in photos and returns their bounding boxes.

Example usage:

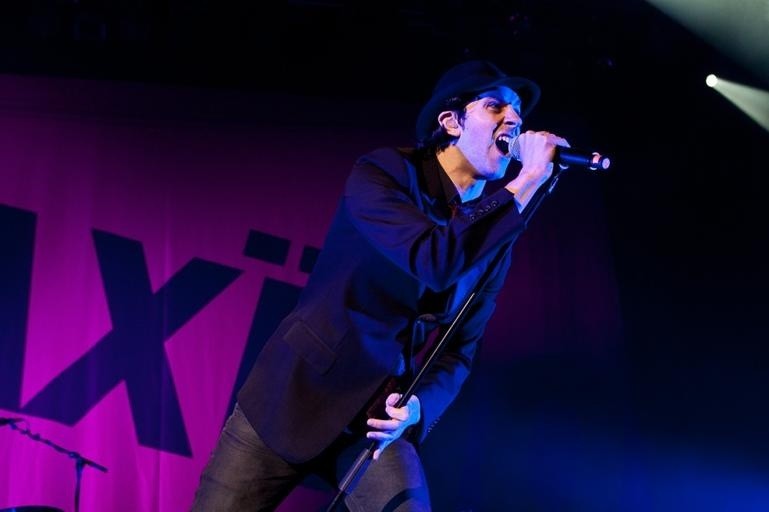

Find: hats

[416,61,540,141]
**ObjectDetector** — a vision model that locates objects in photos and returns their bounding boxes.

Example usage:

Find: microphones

[508,136,611,171]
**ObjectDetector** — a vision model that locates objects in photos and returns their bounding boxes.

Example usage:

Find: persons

[191,58,571,512]
